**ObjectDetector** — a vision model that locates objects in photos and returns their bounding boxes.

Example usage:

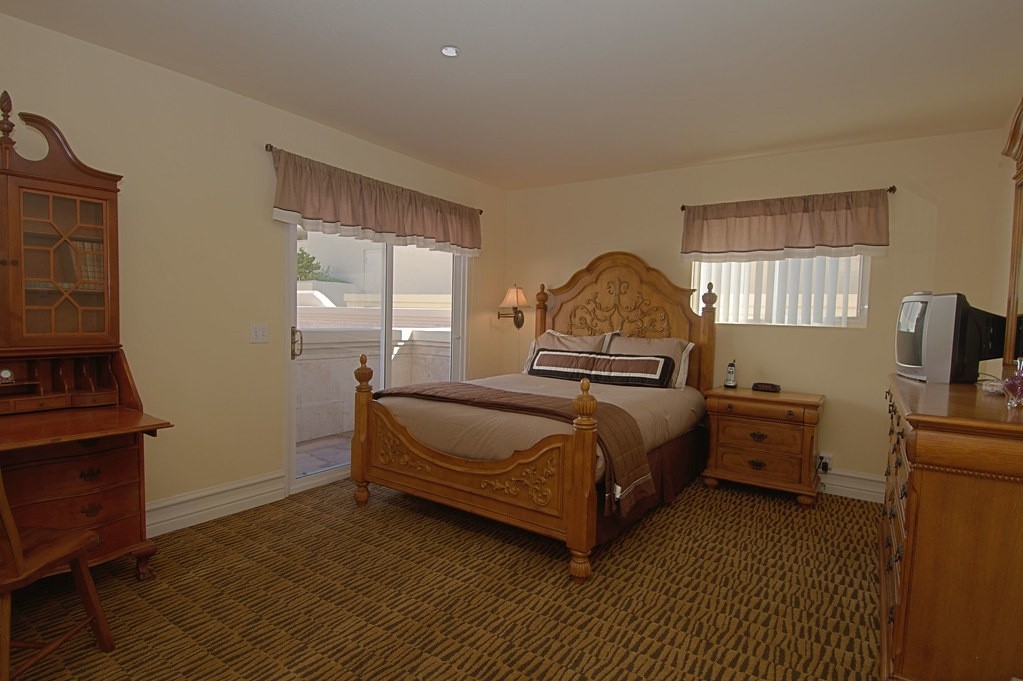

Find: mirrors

[998,93,1023,379]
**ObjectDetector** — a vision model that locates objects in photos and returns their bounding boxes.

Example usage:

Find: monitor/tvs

[895,293,1006,384]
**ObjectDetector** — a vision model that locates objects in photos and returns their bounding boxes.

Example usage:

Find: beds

[352,251,719,582]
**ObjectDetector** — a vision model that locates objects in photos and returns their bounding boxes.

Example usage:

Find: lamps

[498,282,530,328]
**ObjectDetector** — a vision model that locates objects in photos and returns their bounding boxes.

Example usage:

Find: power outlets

[818,454,832,474]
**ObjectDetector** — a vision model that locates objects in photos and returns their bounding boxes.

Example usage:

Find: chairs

[0,472,115,681]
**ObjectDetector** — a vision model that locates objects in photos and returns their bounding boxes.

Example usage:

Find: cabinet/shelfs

[879,372,1023,680]
[1,88,175,589]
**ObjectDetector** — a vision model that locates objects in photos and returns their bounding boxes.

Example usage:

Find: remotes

[913,291,931,295]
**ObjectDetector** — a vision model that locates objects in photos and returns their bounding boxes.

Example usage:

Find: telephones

[724,359,736,388]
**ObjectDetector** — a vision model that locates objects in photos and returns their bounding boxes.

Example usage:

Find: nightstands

[702,386,826,510]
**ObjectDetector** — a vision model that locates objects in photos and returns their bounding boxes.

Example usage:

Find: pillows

[606,330,695,391]
[521,329,612,376]
[527,347,675,388]
